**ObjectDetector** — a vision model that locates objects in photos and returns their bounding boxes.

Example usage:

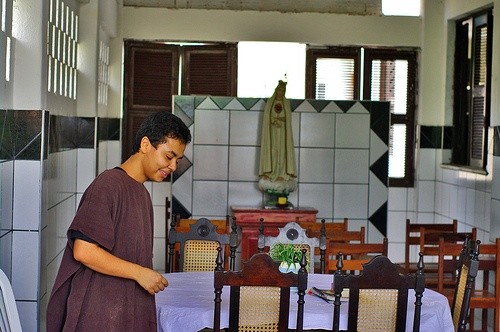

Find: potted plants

[270,241,310,274]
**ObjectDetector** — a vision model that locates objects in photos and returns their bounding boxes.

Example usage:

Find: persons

[259,80,297,181]
[46,112,191,332]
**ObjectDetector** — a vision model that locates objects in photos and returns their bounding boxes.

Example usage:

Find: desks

[155,270,454,332]
[232,205,319,270]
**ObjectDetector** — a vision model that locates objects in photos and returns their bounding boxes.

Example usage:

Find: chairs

[169,218,500,332]
[0,268,22,332]
